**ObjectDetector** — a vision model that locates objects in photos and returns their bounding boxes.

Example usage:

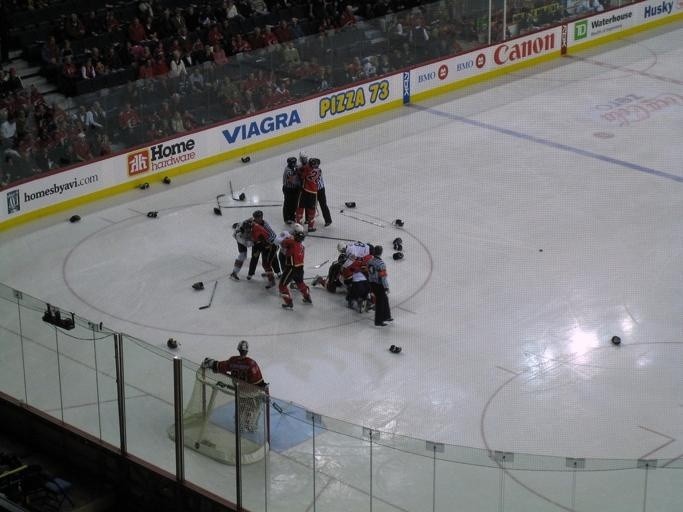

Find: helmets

[290,223,307,242]
[336,241,347,253]
[237,340,249,354]
[286,150,321,168]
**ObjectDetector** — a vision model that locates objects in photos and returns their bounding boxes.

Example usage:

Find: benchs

[48,1,460,180]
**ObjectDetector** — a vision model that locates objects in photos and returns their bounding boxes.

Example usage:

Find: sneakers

[227,269,284,289]
[276,273,322,310]
[343,295,394,328]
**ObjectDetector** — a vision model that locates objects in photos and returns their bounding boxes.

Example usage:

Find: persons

[230,152,395,327]
[202,340,267,434]
[0,0,644,187]
